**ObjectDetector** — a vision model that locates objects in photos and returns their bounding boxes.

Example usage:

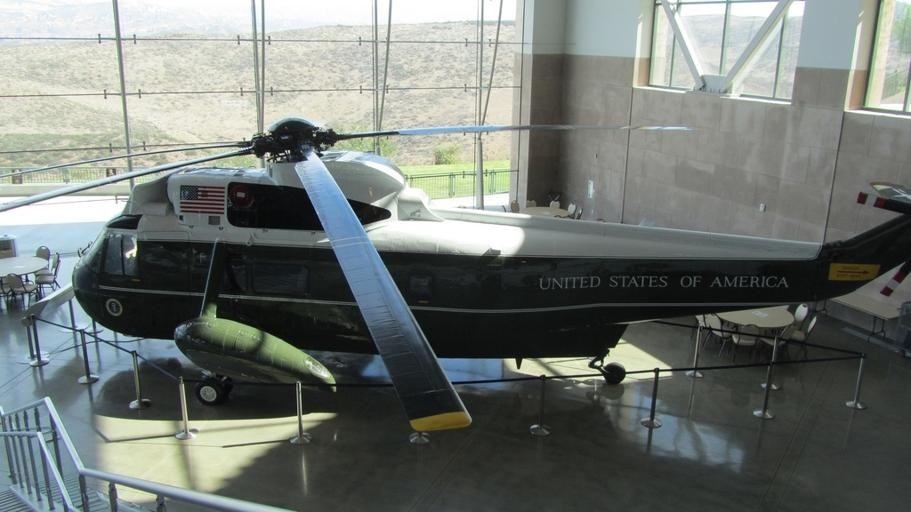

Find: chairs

[0,246,63,309]
[692,303,819,361]
[502,199,583,220]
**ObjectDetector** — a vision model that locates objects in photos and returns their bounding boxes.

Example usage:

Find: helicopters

[0,115,911,437]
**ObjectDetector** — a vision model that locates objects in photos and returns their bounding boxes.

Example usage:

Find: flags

[178,184,225,215]
